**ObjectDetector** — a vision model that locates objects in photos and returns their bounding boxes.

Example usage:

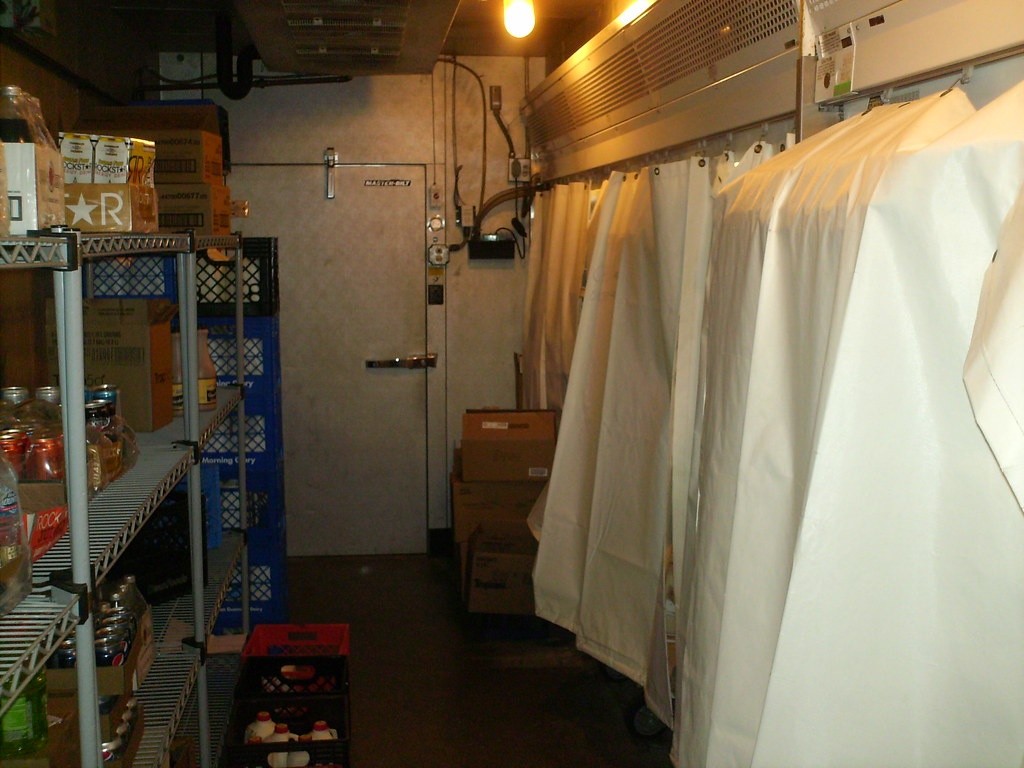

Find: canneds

[57,132,157,186]
[0,383,127,617]
[43,574,150,767]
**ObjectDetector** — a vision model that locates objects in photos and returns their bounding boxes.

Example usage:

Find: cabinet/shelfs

[3,231,257,768]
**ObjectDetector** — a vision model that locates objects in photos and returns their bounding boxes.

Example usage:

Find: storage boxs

[39,300,180,432]
[2,81,67,233]
[57,130,161,235]
[83,96,566,632]
[244,648,351,768]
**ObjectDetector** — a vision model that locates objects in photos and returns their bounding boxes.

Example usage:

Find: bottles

[1,661,50,758]
[243,708,337,768]
[0,82,32,142]
[172,329,184,417]
[196,326,218,410]
[100,572,150,615]
[0,447,35,617]
